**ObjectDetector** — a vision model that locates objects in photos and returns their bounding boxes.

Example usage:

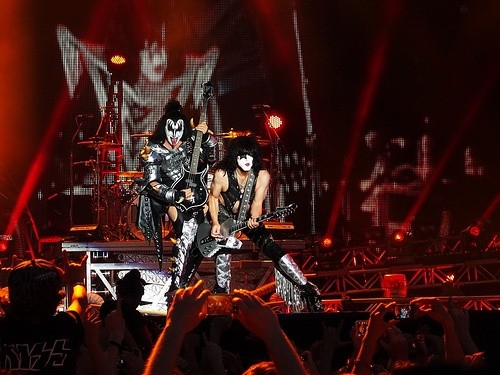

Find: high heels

[301,289,323,312]
[164,288,177,297]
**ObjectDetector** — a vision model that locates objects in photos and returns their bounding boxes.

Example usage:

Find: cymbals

[129,133,153,139]
[215,130,259,138]
[109,168,144,177]
[78,138,124,151]
[74,158,120,165]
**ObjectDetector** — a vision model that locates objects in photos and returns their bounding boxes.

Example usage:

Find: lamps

[383,229,415,266]
[453,224,486,263]
[106,51,127,81]
[310,234,344,273]
[265,111,287,138]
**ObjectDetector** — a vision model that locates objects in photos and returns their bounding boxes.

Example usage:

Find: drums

[166,80,213,213]
[121,197,175,240]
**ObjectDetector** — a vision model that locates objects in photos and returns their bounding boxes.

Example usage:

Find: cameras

[394,307,418,318]
[202,291,237,316]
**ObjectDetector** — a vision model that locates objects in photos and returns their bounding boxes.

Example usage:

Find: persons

[0,259,500,375]
[207,137,323,311]
[134,106,219,287]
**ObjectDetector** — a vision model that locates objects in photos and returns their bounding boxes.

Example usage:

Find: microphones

[76,113,95,118]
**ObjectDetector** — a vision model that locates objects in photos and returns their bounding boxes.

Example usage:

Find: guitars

[196,203,297,259]
